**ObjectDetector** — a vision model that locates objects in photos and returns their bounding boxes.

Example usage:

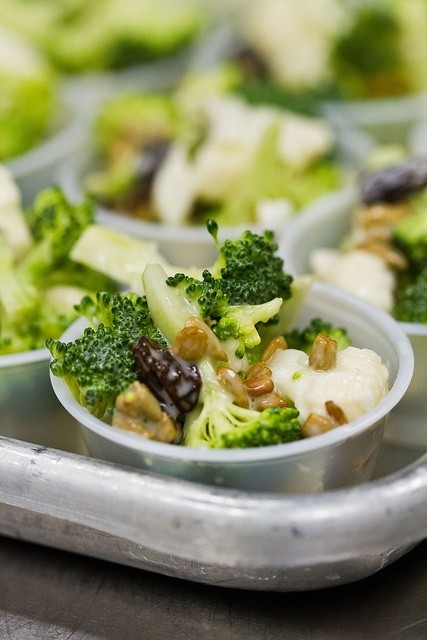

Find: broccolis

[387,212,427,325]
[2,165,122,358]
[49,221,348,450]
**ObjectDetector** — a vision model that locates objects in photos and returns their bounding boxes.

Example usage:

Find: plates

[1,397,427,591]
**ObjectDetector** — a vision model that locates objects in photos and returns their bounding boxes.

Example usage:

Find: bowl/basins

[221,3,427,132]
[273,184,427,394]
[58,130,378,266]
[48,279,415,495]
[0,79,88,201]
[1,1,218,97]
[406,119,427,163]
[1,245,166,417]
